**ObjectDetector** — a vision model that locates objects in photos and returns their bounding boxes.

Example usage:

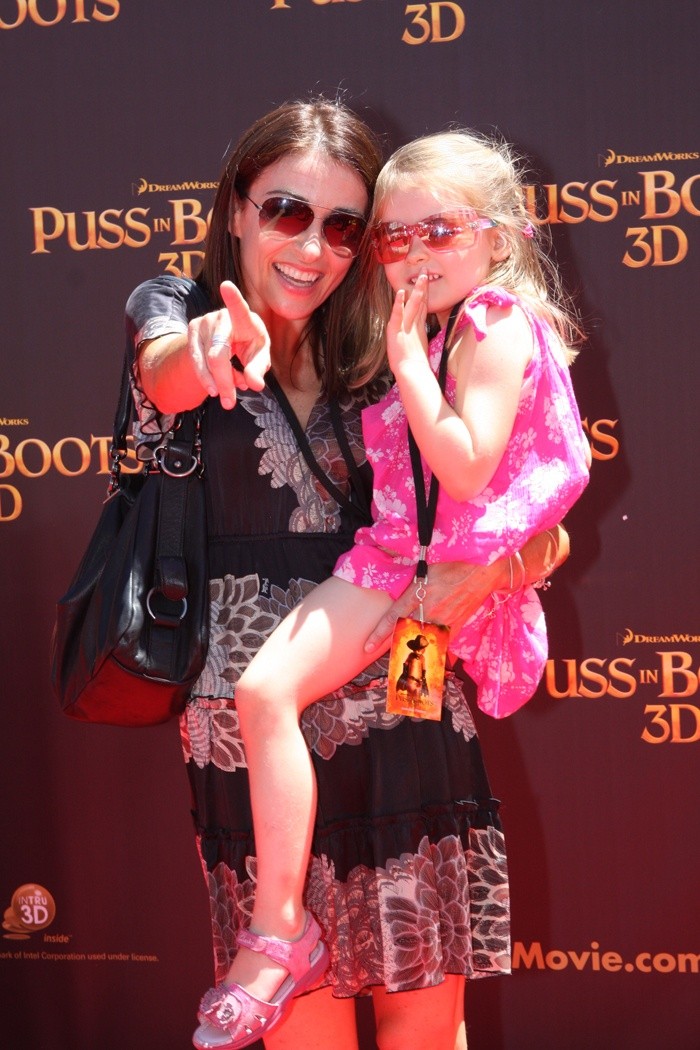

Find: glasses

[245,196,368,259]
[370,209,499,265]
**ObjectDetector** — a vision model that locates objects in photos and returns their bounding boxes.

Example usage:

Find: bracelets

[528,529,562,591]
[487,550,527,620]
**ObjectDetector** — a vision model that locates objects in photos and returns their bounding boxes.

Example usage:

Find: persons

[122,100,513,1050]
[194,122,599,1050]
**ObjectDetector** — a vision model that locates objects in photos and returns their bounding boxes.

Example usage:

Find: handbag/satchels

[51,273,210,729]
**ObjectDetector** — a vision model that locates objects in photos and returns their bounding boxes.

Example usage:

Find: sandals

[192,910,330,1050]
